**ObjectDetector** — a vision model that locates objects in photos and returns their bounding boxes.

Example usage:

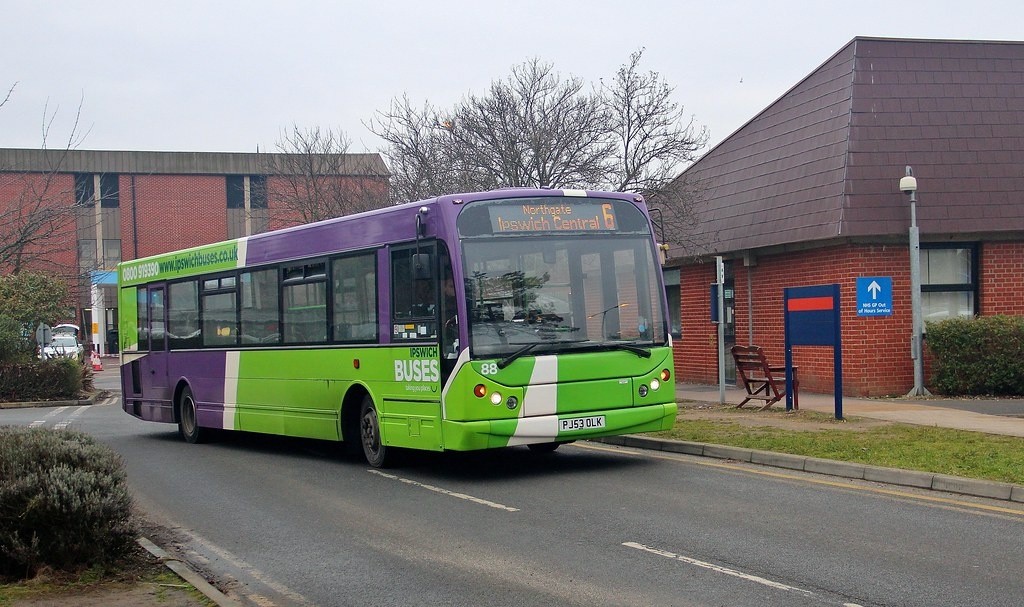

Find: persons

[435,267,461,351]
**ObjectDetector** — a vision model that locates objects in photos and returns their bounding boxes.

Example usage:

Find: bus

[116,184,678,468]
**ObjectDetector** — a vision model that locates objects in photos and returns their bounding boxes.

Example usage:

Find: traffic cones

[89,351,104,371]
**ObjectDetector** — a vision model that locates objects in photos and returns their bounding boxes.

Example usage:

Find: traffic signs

[856,276,893,317]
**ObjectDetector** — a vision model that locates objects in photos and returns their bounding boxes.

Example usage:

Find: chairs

[730,344,800,411]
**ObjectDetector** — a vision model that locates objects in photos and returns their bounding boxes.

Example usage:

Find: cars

[37,336,86,366]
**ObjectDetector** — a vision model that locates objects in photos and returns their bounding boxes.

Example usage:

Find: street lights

[586,303,630,343]
